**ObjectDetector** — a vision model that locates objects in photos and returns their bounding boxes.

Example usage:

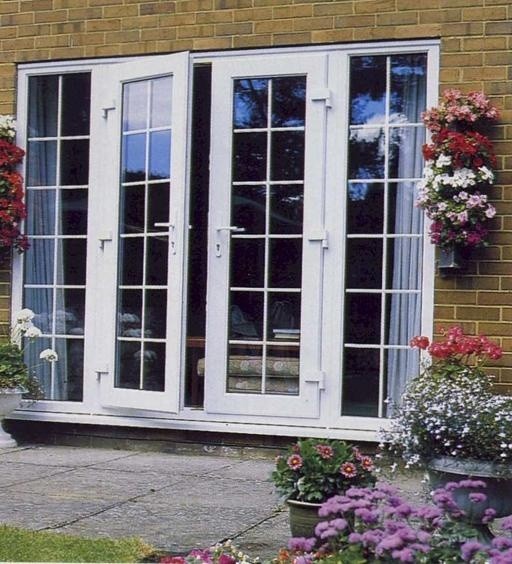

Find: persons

[228,241,298,358]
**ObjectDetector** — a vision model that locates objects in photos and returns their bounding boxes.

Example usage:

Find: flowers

[412,87,503,247]
[0,113,33,259]
[270,435,377,503]
[379,327,510,464]
[0,307,65,408]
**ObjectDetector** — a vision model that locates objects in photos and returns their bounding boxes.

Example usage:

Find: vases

[426,454,510,524]
[1,388,25,450]
[285,499,357,545]
[440,247,466,272]
